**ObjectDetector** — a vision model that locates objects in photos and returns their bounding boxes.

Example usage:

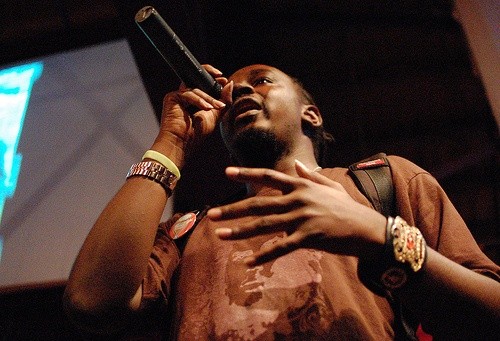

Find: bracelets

[357,214,427,296]
[125,163,180,196]
[141,150,183,182]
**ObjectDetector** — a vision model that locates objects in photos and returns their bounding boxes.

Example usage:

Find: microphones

[135,6,223,103]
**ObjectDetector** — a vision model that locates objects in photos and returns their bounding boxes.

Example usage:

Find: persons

[60,62,499,338]
